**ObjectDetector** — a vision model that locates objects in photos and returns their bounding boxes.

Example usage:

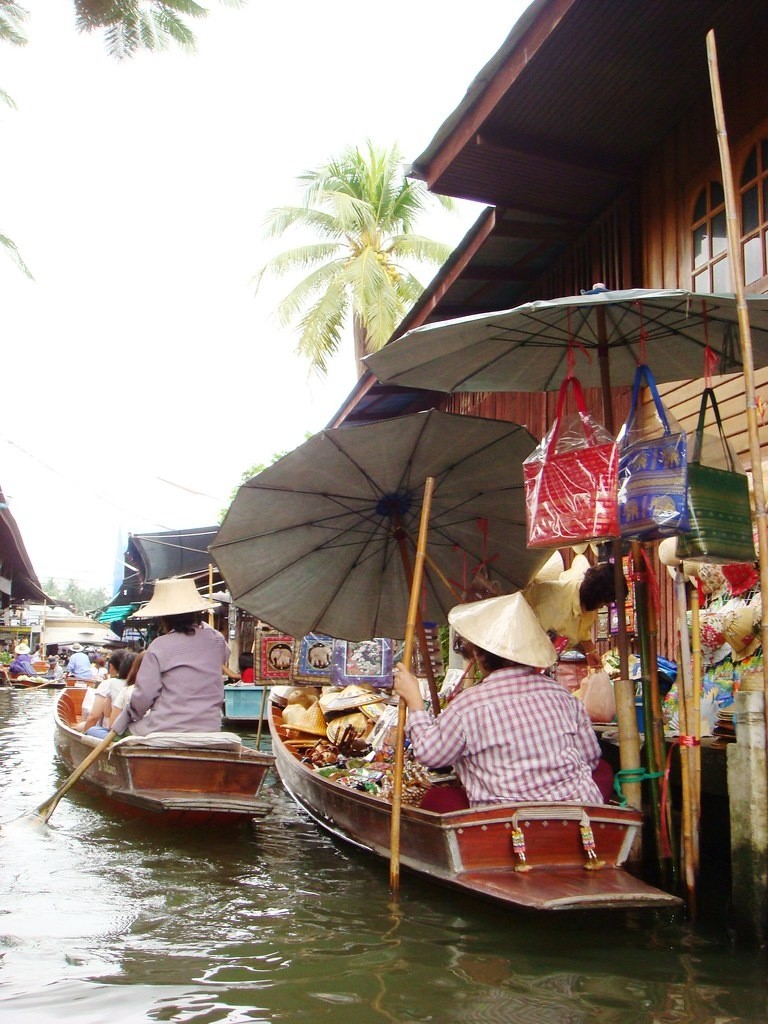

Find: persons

[8,643,31,672]
[520,563,628,670]
[32,643,112,682]
[223,652,253,683]
[395,591,612,814]
[114,578,231,738]
[77,650,151,739]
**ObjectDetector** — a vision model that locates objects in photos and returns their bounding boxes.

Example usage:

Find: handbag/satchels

[522,378,620,549]
[676,389,758,563]
[618,365,693,540]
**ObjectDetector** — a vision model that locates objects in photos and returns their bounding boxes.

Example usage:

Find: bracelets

[586,650,597,655]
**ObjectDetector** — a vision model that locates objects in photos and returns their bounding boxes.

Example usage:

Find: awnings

[0,486,62,606]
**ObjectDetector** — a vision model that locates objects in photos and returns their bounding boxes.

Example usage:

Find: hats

[559,554,591,581]
[536,550,564,581]
[658,537,689,583]
[55,655,60,659]
[47,656,57,665]
[270,683,426,748]
[722,563,759,596]
[448,592,557,668]
[658,593,763,666]
[133,579,222,617]
[69,643,84,652]
[682,561,700,588]
[15,643,31,654]
[698,562,730,600]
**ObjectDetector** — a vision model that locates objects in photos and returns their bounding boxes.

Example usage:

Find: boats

[51,684,280,826]
[219,684,275,720]
[0,659,102,690]
[265,683,686,913]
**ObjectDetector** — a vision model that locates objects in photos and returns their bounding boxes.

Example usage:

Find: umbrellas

[203,593,233,604]
[361,284,767,681]
[207,407,554,717]
